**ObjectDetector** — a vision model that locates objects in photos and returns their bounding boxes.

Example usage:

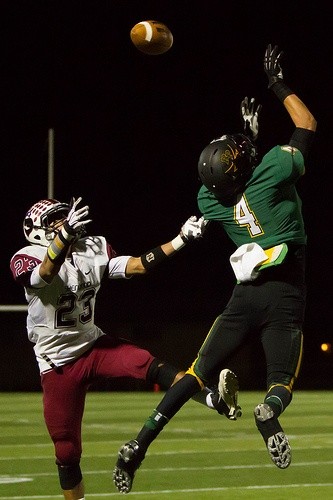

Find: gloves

[240,97,263,142]
[61,196,94,242]
[180,215,210,245]
[263,43,287,88]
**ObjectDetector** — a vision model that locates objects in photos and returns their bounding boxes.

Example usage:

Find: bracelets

[172,235,186,251]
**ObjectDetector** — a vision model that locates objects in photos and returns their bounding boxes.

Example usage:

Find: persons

[113,44,318,495]
[10,197,242,500]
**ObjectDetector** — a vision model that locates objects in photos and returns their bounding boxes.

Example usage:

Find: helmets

[198,132,257,196]
[22,198,72,248]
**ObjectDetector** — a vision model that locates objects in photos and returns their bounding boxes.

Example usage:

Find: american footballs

[130,20,174,54]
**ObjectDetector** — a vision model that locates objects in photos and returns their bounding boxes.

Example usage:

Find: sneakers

[253,403,293,469]
[112,439,147,494]
[209,368,243,421]
